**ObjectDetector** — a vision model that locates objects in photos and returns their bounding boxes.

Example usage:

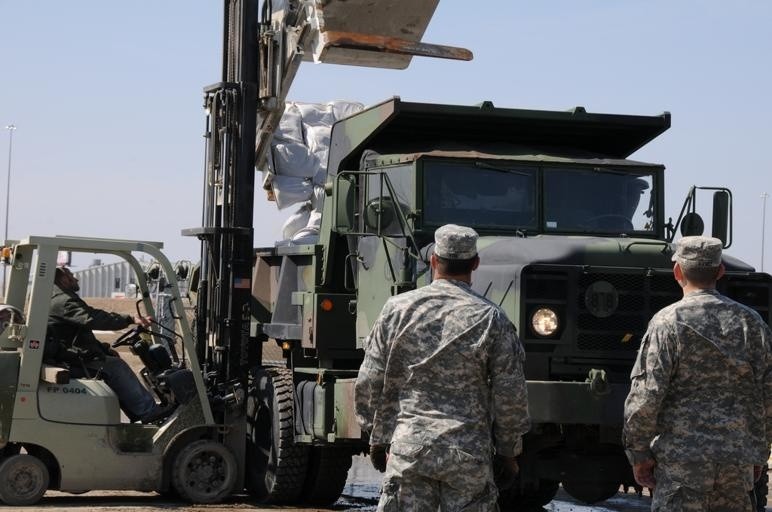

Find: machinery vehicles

[0,1,476,506]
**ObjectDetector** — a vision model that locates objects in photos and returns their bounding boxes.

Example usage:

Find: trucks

[184,89,770,504]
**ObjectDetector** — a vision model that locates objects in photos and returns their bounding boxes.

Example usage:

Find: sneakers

[141,404,175,424]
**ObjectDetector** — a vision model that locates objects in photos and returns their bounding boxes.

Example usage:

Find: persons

[619,232,772,512]
[352,223,532,512]
[40,263,174,425]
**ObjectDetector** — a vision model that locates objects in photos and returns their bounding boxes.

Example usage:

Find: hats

[434,224,479,261]
[671,236,723,267]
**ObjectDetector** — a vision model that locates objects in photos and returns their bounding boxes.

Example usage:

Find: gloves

[494,455,519,490]
[369,447,386,472]
